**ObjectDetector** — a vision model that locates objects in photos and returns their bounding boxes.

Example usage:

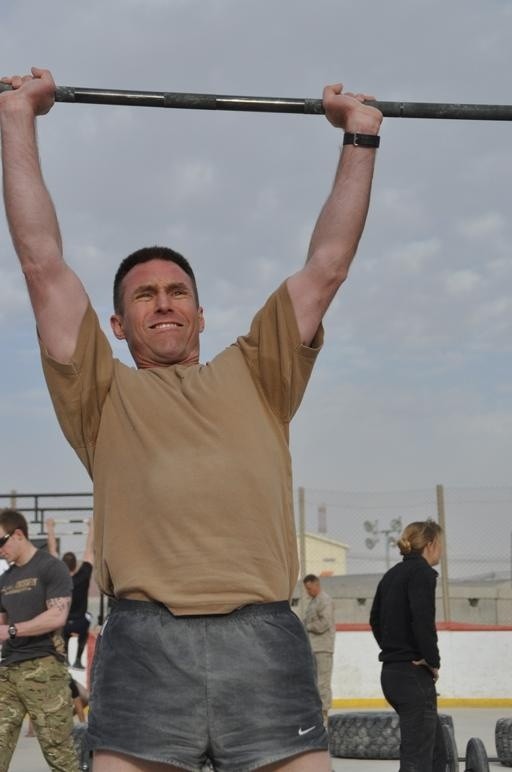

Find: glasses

[0,532,13,548]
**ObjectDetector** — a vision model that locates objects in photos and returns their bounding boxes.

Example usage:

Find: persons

[24,675,86,736]
[369,520,447,770]
[0,66,383,771]
[46,518,95,671]
[1,510,83,771]
[301,574,336,727]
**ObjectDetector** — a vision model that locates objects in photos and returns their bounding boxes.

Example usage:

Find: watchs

[9,624,18,640]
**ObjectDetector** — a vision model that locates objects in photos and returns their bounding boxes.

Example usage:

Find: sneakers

[73,660,85,670]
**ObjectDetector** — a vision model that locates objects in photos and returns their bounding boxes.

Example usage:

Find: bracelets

[343,134,380,150]
